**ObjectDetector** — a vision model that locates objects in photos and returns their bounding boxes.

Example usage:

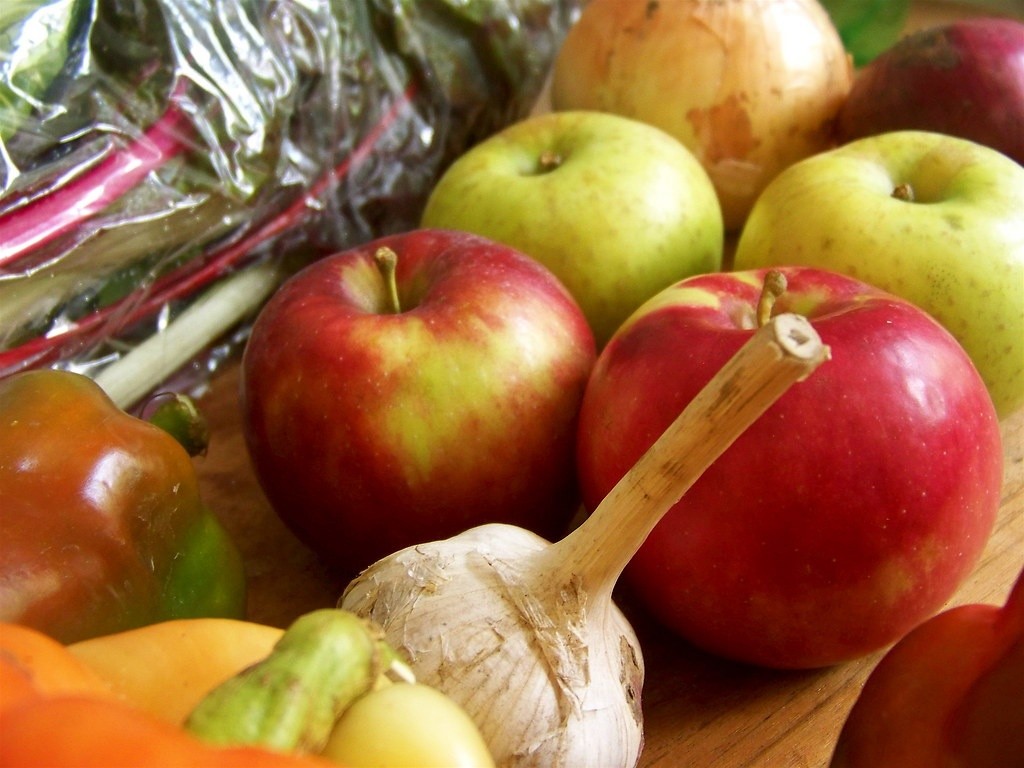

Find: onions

[554,0,861,231]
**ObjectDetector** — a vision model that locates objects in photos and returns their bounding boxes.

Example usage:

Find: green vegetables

[0,0,564,446]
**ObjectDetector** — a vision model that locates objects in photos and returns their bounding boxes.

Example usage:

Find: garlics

[328,311,825,768]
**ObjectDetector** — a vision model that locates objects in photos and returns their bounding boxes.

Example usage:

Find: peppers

[0,368,510,768]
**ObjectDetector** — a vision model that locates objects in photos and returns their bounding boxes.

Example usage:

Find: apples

[243,110,1024,669]
